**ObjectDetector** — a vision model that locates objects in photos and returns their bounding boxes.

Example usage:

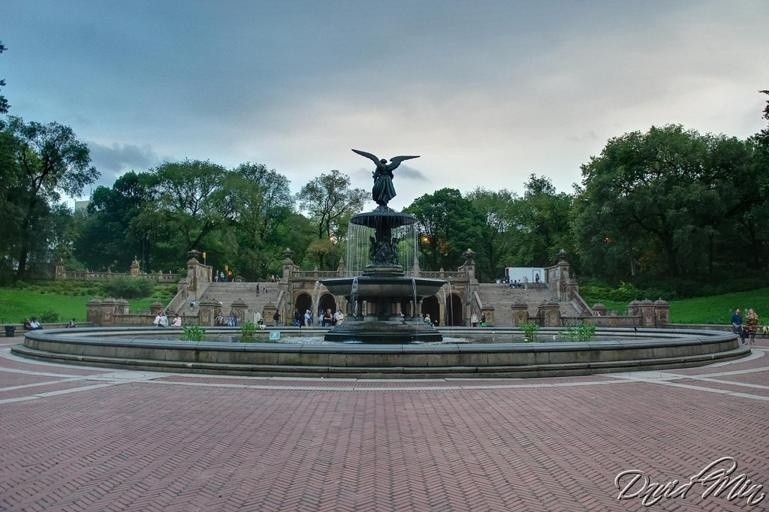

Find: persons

[158,313,169,327]
[256,283,260,296]
[295,308,344,328]
[351,148,420,208]
[746,308,759,344]
[470,311,478,327]
[495,275,528,290]
[30,316,43,330]
[479,311,486,327]
[536,273,539,281]
[152,312,162,326]
[424,313,432,326]
[212,267,236,283]
[368,237,403,265]
[66,316,76,328]
[170,313,182,327]
[731,307,748,345]
[276,310,280,326]
[188,296,196,313]
[24,318,32,330]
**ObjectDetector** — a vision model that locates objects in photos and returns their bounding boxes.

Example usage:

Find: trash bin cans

[5,326,16,337]
